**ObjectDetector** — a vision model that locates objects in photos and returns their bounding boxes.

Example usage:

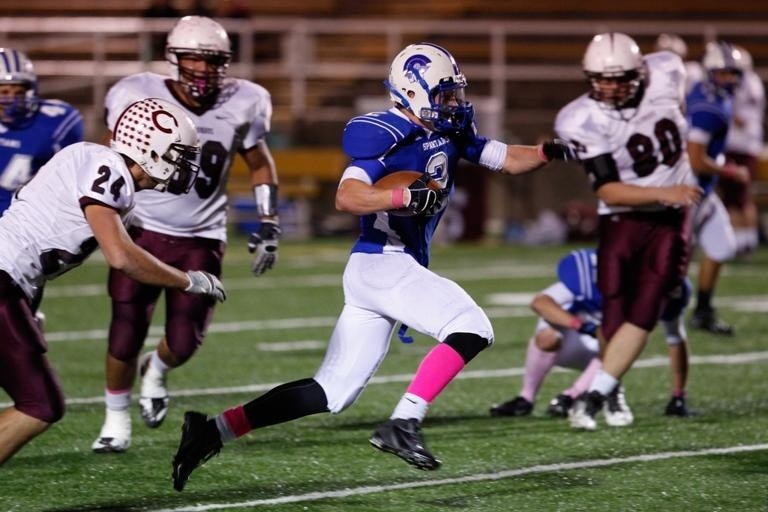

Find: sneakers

[92,409,132,452]
[172,411,222,492]
[139,350,169,428]
[689,306,732,333]
[370,418,439,470]
[491,386,685,430]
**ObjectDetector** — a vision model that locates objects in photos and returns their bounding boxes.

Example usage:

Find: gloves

[185,270,226,302]
[406,173,449,214]
[248,220,281,274]
[539,139,586,162]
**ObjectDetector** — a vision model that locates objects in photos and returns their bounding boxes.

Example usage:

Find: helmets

[582,32,643,106]
[705,46,751,76]
[383,42,473,130]
[0,48,38,118]
[110,98,200,195]
[166,16,232,96]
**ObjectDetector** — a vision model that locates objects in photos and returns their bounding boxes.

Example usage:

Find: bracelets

[672,389,687,398]
[392,187,405,209]
[251,183,279,219]
[538,144,549,163]
[570,316,583,330]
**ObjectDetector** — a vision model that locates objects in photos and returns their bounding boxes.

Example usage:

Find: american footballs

[373,171,442,216]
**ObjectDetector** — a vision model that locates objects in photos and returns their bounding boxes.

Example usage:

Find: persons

[91,13,281,454]
[0,98,227,466]
[553,32,705,431]
[488,247,693,418]
[657,34,768,336]
[1,47,85,220]
[172,42,587,492]
